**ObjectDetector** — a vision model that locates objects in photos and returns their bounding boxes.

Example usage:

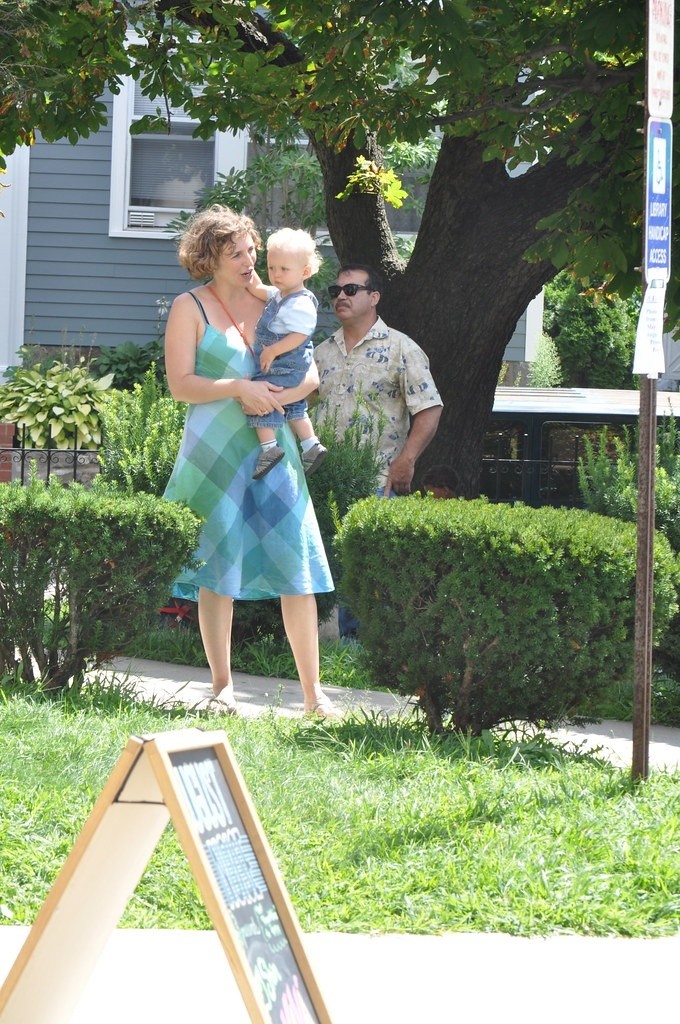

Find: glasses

[328,283,369,297]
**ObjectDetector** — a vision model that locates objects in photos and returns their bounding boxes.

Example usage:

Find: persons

[164,202,345,725]
[419,463,458,501]
[271,263,445,670]
[245,227,327,481]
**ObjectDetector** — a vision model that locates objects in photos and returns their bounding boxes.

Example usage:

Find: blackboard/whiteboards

[142,728,334,1024]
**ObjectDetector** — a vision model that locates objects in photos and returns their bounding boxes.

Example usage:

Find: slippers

[207,698,236,715]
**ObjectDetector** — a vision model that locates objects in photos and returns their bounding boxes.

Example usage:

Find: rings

[262,410,268,415]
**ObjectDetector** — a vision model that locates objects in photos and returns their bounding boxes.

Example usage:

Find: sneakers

[252,445,285,479]
[301,443,328,478]
